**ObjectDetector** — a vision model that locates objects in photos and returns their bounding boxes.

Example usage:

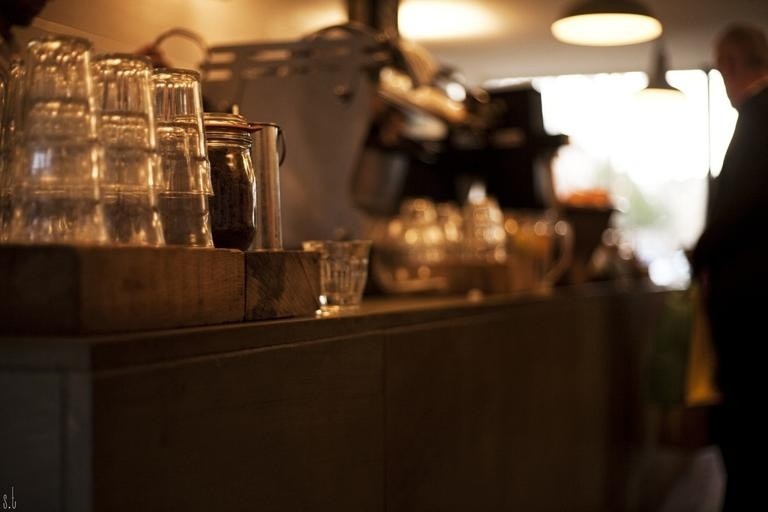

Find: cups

[299,238,373,314]
[0,32,111,250]
[382,186,618,287]
[152,68,215,251]
[97,53,167,247]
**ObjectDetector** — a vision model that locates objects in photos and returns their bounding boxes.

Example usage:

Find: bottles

[202,110,263,250]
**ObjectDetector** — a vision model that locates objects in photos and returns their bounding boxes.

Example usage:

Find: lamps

[549,1,664,48]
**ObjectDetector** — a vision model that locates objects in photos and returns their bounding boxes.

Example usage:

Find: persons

[687,15,768,510]
